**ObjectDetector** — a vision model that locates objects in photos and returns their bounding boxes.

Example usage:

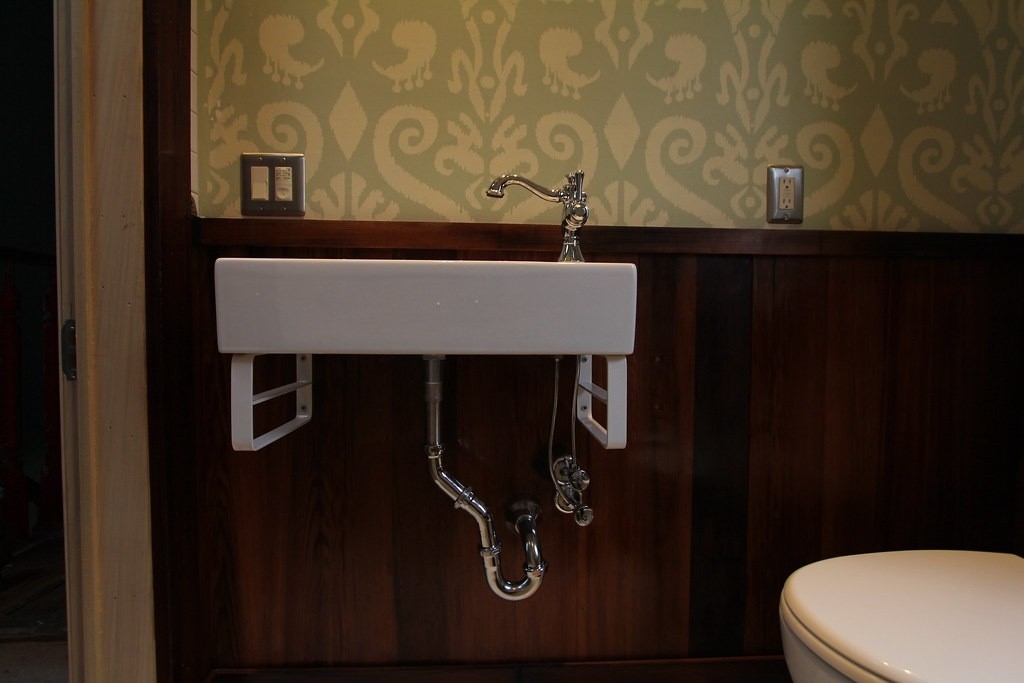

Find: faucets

[485,168,589,244]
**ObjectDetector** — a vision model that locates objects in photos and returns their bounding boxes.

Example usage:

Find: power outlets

[768,164,805,224]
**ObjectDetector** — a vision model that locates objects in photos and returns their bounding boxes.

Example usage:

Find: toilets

[778,549,1024,682]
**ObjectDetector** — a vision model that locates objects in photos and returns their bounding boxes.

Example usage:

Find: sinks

[214,257,638,356]
